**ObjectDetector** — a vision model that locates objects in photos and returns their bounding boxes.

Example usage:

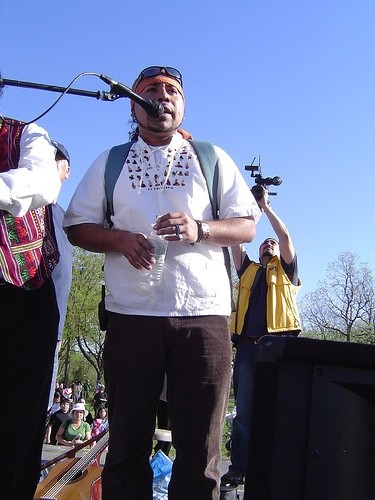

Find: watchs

[195,219,210,243]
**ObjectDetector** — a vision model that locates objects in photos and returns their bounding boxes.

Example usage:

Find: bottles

[71,433,82,445]
[139,214,169,289]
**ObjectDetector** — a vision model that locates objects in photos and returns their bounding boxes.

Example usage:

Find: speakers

[243,335,375,500]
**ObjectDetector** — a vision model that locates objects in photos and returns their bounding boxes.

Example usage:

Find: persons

[154,372,176,455]
[44,377,108,448]
[0,75,61,500]
[62,66,264,500]
[47,140,72,411]
[221,186,302,490]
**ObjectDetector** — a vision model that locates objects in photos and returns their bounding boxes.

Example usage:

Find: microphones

[100,74,164,118]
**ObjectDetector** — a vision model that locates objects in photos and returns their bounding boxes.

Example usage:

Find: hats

[130,66,186,110]
[51,140,71,164]
[70,403,88,417]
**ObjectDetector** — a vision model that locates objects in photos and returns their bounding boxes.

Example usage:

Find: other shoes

[220,470,245,491]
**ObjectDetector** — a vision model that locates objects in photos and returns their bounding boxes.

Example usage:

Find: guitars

[33,430,109,500]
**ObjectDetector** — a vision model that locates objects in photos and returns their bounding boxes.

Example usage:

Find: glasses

[133,66,183,92]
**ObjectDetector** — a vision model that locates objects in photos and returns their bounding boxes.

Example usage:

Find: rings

[176,225,180,235]
